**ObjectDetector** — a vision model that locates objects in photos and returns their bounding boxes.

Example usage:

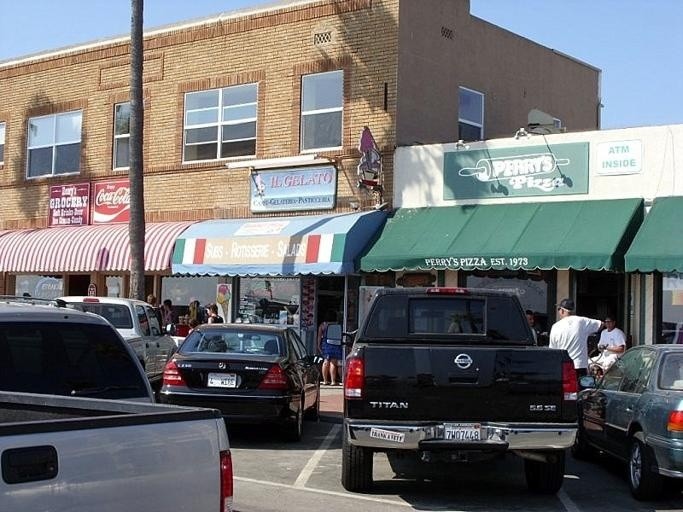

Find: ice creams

[218,285,231,323]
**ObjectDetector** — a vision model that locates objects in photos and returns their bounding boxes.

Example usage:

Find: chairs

[208,340,227,352]
[264,340,277,354]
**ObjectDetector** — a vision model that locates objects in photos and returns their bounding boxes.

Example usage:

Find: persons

[316,310,341,386]
[547,299,605,392]
[187,319,198,334]
[146,293,156,306]
[524,309,538,346]
[204,303,223,324]
[585,317,626,374]
[162,299,178,326]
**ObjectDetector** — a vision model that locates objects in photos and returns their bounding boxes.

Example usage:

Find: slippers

[321,381,340,386]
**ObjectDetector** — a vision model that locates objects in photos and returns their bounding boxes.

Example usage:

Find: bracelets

[604,343,608,349]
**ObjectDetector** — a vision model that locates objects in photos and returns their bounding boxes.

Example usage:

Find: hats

[554,298,575,312]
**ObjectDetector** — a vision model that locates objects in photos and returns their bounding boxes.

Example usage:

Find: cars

[578,343,683,501]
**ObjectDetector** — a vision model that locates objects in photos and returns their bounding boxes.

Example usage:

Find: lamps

[514,126,528,140]
[455,139,466,151]
[247,166,257,178]
